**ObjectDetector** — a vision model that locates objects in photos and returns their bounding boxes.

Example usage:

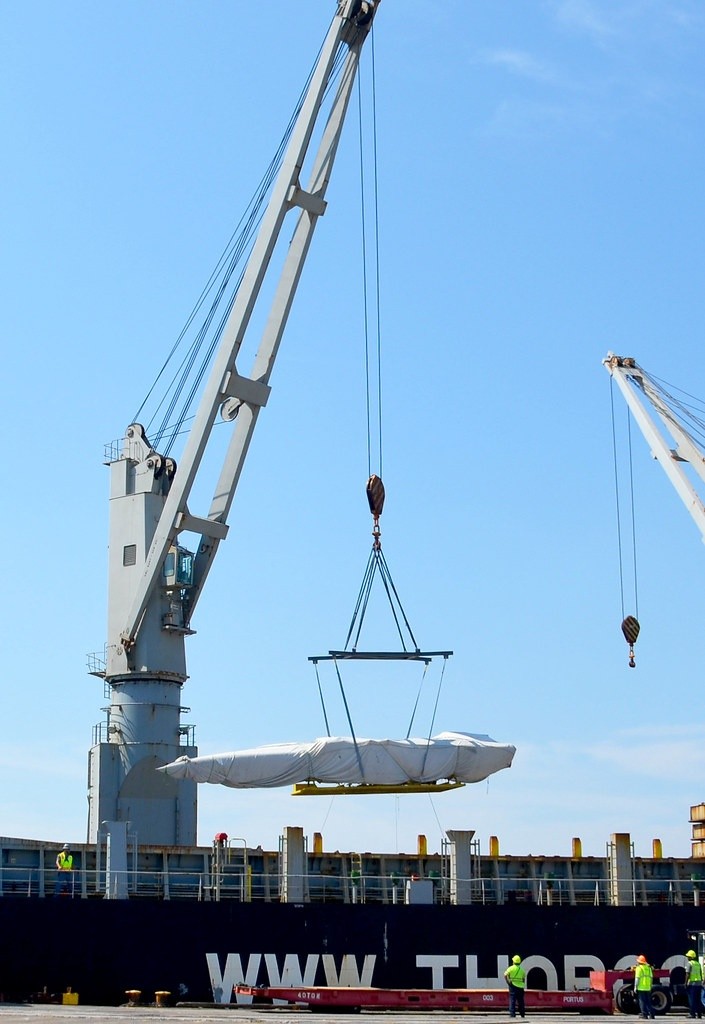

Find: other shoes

[637,1015,649,1020]
[686,1013,696,1019]
[649,1015,656,1019]
[698,1015,702,1019]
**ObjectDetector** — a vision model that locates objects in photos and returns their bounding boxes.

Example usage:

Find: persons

[684,949,705,1019]
[53,844,74,900]
[503,955,527,1017]
[634,955,654,1019]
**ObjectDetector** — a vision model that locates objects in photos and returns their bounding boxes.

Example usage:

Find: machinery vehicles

[84,0,397,890]
[599,351,705,672]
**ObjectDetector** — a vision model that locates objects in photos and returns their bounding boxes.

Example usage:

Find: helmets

[685,950,697,958]
[636,955,646,964]
[63,844,71,850]
[511,955,521,964]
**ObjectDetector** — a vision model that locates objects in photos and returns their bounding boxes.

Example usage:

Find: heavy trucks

[231,929,705,1014]
